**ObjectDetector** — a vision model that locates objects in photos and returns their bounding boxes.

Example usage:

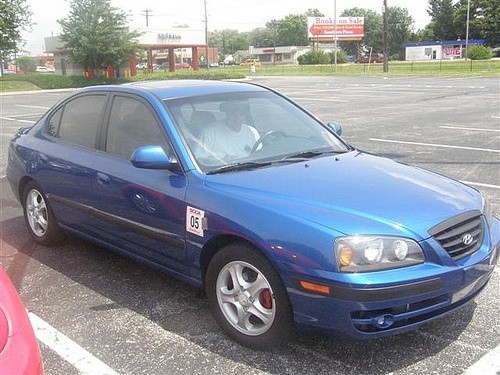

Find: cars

[34,65,54,72]
[240,58,262,67]
[7,80,500,352]
[137,60,240,70]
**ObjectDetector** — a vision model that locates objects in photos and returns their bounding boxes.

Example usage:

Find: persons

[195,100,263,164]
[121,105,171,158]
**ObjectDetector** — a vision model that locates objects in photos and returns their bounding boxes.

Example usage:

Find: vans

[354,53,383,63]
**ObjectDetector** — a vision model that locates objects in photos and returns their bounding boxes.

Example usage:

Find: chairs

[120,110,217,158]
